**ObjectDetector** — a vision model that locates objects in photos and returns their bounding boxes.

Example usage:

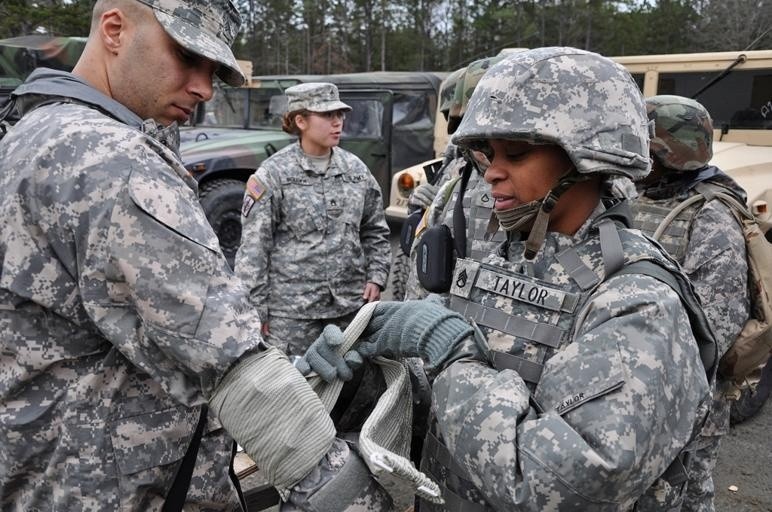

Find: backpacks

[651,187,772,402]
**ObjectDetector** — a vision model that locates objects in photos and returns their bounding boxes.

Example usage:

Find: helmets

[447,56,506,135]
[646,94,714,171]
[450,45,654,182]
[439,66,468,112]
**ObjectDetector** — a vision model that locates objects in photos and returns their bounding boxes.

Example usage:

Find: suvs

[0,35,89,114]
[384,50,772,426]
[178,71,454,270]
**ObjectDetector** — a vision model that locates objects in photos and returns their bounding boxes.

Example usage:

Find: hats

[137,0,247,87]
[285,82,352,113]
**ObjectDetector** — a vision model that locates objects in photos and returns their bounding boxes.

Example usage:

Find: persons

[406,67,466,216]
[0,0,389,512]
[631,95,767,512]
[295,46,719,512]
[405,56,512,300]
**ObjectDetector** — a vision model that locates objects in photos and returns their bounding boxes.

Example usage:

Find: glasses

[303,110,346,121]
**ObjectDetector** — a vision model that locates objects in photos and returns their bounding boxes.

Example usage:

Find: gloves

[355,292,476,372]
[407,183,440,210]
[295,323,364,386]
